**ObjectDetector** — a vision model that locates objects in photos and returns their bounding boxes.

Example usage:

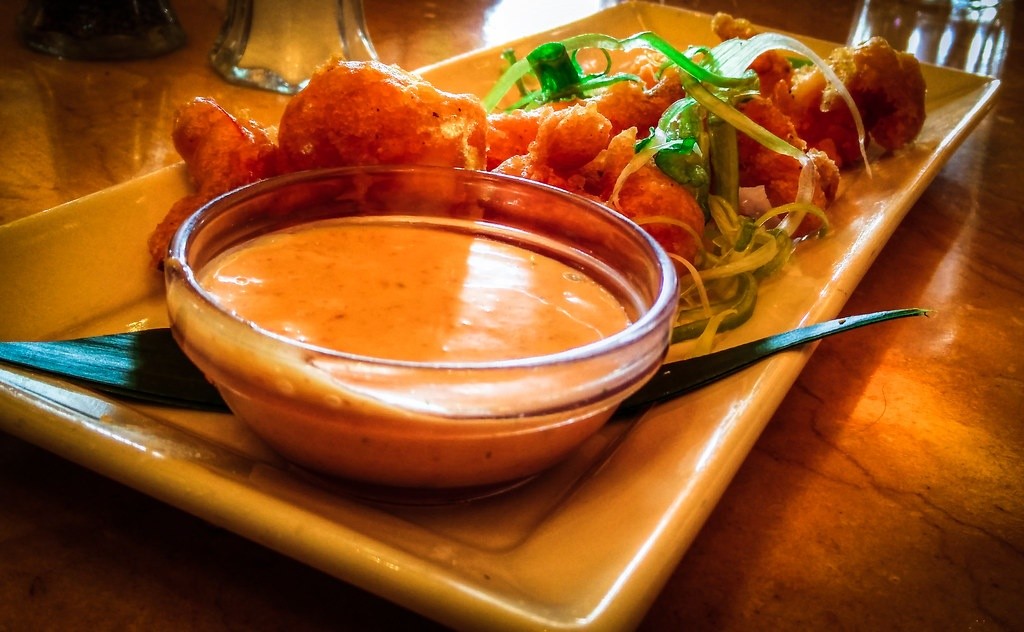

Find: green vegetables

[474,32,871,355]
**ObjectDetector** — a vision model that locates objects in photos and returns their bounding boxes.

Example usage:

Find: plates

[0,0,1005,626]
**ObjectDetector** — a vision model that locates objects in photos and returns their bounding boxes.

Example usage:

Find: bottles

[209,1,377,94]
[17,0,186,60]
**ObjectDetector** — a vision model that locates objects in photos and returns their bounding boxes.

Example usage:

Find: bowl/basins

[166,169,678,491]
